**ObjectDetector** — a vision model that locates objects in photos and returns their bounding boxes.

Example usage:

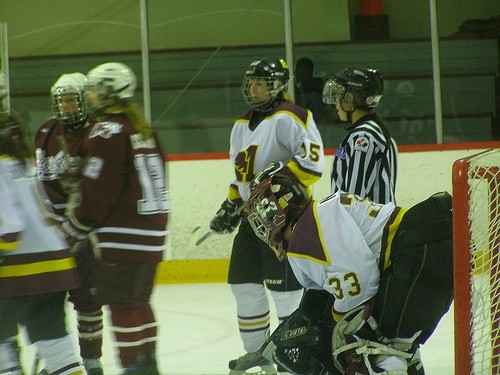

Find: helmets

[52,72,88,101]
[246,57,290,91]
[84,61,138,98]
[332,65,385,110]
[248,176,311,244]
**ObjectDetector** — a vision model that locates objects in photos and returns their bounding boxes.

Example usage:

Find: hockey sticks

[194,200,250,246]
[57,133,102,264]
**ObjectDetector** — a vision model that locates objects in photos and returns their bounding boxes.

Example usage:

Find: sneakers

[228,351,277,375]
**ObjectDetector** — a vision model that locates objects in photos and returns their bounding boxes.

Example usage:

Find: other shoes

[83,357,104,375]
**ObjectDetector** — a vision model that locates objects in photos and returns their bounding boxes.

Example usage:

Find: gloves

[209,197,245,234]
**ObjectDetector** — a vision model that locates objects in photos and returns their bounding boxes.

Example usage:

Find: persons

[247,175,455,375]
[208,56,325,372]
[0,110,88,375]
[35,71,105,375]
[59,63,169,375]
[323,65,399,208]
[294,56,326,121]
